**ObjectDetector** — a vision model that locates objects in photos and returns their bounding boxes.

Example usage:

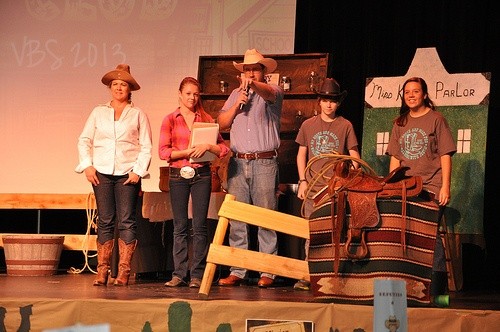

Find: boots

[113,238,138,286]
[93,238,115,286]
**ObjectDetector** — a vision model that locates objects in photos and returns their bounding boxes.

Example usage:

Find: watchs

[298,179,306,185]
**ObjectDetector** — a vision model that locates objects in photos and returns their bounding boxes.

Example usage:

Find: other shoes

[294,280,310,291]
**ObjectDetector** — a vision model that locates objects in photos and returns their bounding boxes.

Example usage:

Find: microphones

[240,85,251,110]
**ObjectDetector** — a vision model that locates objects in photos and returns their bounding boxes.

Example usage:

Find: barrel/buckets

[2,235,65,276]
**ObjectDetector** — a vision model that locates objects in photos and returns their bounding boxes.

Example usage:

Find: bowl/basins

[278,183,299,194]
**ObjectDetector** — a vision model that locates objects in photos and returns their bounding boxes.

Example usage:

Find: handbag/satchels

[307,161,443,304]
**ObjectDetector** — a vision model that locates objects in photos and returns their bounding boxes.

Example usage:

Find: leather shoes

[218,274,249,287]
[257,276,275,288]
[164,275,188,287]
[189,277,201,288]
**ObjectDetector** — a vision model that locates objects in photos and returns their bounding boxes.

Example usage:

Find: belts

[170,165,210,180]
[238,151,276,161]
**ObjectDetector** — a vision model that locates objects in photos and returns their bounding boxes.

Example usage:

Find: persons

[216,49,289,289]
[73,65,152,286]
[386,77,457,307]
[159,76,230,289]
[295,78,360,290]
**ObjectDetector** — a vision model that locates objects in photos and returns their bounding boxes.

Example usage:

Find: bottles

[294,110,319,131]
[279,77,290,91]
[218,80,228,93]
[307,71,319,92]
[210,165,222,193]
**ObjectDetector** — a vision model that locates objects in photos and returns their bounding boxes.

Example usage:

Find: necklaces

[320,115,332,146]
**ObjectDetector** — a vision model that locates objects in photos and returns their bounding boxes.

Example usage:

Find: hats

[233,49,277,74]
[101,64,140,91]
[313,78,348,99]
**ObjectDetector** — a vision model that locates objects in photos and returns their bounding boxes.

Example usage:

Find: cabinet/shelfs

[197,51,338,184]
